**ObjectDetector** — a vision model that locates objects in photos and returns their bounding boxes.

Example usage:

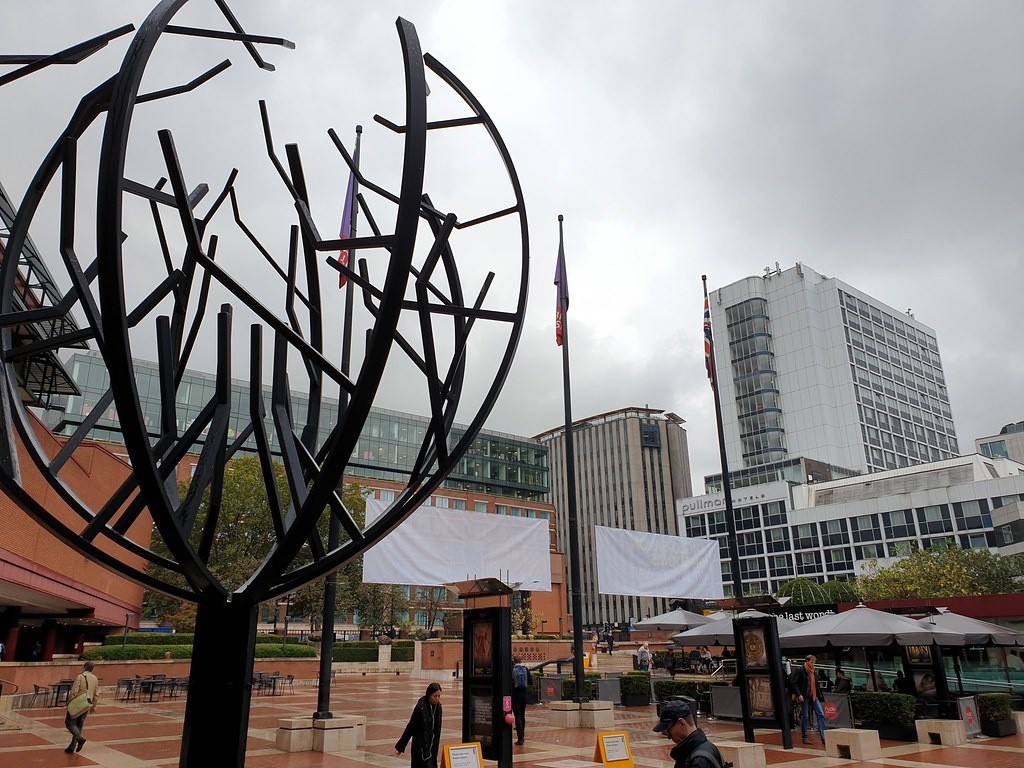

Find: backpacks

[512,665,528,688]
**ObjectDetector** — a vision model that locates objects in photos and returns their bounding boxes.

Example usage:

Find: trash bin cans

[583,651,591,669]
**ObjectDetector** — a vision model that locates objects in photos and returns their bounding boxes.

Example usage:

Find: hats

[652,700,690,732]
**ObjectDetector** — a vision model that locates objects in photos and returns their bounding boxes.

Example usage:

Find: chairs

[28,679,74,709]
[113,673,191,703]
[686,657,714,676]
[250,671,295,696]
[315,671,337,687]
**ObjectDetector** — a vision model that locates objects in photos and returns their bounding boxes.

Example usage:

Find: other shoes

[822,740,825,744]
[802,738,813,744]
[515,741,523,745]
[65,747,74,753]
[76,738,86,752]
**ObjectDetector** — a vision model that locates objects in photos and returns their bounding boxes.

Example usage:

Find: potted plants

[850,691,920,742]
[653,680,695,717]
[977,693,1017,739]
[617,674,650,707]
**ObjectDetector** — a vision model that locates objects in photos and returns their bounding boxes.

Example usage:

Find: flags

[703,288,716,391]
[553,223,570,345]
[337,137,360,289]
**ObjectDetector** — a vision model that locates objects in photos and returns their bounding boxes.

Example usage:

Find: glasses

[664,720,678,736]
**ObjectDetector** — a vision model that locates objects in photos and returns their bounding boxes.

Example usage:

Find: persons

[866,669,908,693]
[32,639,42,660]
[592,623,615,655]
[663,649,676,680]
[689,645,731,675]
[0,640,6,662]
[781,667,852,730]
[662,700,726,768]
[795,655,827,744]
[566,629,571,636]
[65,662,99,754]
[637,642,650,670]
[1001,649,1024,671]
[394,682,443,768]
[512,655,534,745]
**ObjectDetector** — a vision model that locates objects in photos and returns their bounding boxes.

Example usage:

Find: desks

[312,670,336,688]
[676,657,692,675]
[146,673,163,694]
[265,676,284,696]
[163,677,183,698]
[254,672,269,690]
[142,680,164,703]
[48,684,71,708]
[118,678,140,700]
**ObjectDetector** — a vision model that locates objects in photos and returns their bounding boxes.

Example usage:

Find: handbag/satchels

[68,692,93,718]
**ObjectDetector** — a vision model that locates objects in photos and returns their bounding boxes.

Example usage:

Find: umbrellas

[632,607,1024,647]
[661,644,682,649]
